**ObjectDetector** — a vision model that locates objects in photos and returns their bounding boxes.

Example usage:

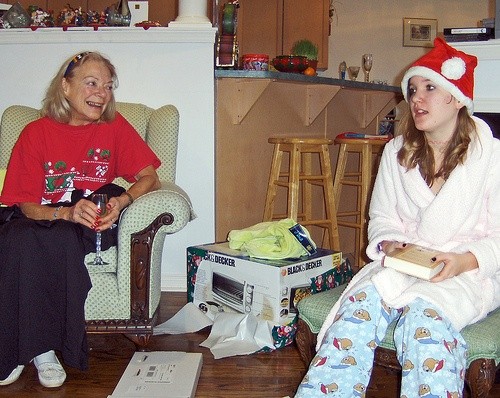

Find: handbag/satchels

[81,183,126,249]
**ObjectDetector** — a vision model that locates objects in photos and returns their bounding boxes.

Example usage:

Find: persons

[296,38,500,398]
[0,51,162,388]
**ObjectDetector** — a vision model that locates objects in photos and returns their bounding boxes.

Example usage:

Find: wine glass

[349,66,360,81]
[362,53,373,83]
[86,193,109,265]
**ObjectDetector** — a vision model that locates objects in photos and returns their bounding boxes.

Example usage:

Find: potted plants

[291,39,318,74]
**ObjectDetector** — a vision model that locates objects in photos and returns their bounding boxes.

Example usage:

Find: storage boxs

[187,242,342,349]
[113,351,203,398]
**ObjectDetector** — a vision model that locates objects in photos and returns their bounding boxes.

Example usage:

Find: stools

[333,139,386,268]
[263,138,339,250]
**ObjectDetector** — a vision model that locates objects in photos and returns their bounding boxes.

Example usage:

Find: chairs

[293,282,500,398]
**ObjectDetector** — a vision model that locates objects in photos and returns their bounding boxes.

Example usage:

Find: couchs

[0,106,196,354]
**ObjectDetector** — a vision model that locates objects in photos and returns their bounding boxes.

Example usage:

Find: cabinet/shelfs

[207,0,328,71]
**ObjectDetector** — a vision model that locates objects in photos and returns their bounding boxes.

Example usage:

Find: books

[383,243,446,280]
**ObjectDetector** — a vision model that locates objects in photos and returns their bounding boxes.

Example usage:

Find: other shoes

[0,365,25,385]
[34,350,66,388]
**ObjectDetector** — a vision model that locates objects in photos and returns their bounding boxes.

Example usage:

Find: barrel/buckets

[242,53,270,71]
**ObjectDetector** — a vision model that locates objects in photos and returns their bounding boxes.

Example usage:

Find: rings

[81,211,84,217]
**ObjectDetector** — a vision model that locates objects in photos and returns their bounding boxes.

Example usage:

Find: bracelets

[121,192,134,205]
[54,206,63,219]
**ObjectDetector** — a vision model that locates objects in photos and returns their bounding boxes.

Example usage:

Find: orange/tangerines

[303,67,314,76]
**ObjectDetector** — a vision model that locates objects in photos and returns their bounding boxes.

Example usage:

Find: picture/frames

[403,18,438,47]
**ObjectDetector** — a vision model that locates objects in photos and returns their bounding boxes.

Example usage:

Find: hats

[400,37,477,116]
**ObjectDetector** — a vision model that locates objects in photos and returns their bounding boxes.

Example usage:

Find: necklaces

[427,139,452,145]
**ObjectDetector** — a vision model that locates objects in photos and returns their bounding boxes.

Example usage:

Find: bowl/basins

[271,55,310,73]
